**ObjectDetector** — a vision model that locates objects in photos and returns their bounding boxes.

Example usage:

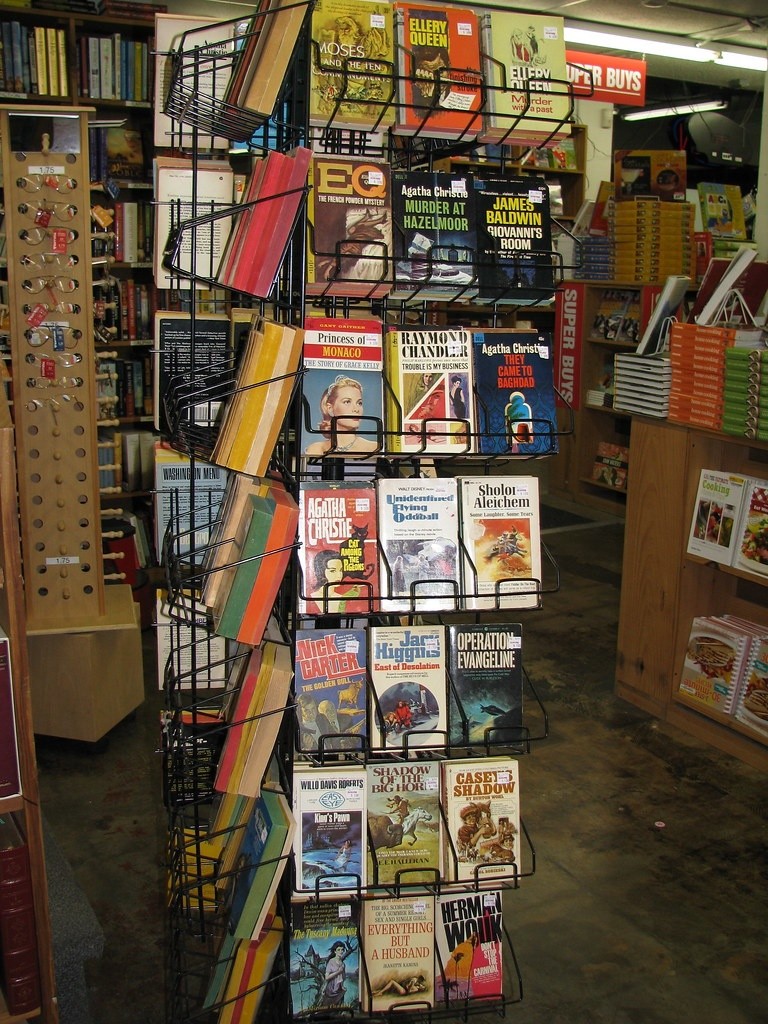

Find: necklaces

[332,440,356,452]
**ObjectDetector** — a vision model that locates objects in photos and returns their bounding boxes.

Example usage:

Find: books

[0,0,167,593]
[575,181,768,736]
[169,0,570,1024]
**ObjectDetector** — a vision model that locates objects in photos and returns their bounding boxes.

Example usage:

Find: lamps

[622,96,728,121]
[563,19,768,72]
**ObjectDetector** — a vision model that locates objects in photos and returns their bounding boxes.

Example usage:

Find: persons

[306,376,378,457]
[318,700,360,749]
[297,694,339,754]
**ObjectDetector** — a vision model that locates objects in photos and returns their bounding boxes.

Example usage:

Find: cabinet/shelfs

[0,0,768,1024]
